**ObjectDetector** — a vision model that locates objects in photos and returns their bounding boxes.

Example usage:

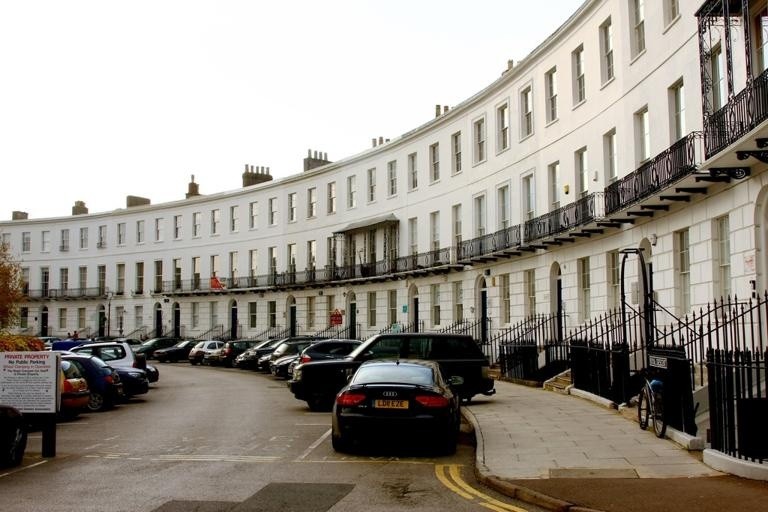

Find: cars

[0,405,28,470]
[328,351,467,461]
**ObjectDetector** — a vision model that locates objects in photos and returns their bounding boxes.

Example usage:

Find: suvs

[285,330,500,413]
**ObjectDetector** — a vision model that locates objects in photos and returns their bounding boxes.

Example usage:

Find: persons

[67,331,79,340]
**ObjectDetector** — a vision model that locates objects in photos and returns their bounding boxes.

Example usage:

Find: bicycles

[629,365,670,440]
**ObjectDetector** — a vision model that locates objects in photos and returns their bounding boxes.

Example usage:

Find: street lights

[105,291,114,337]
